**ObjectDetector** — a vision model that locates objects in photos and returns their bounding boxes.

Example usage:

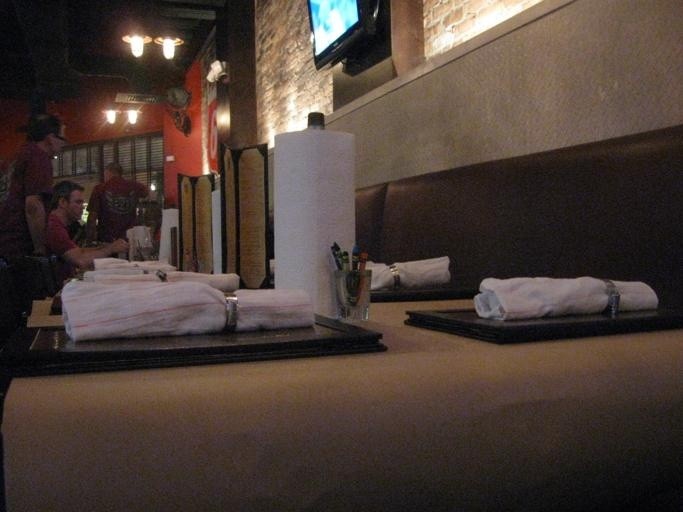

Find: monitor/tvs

[305,0,380,73]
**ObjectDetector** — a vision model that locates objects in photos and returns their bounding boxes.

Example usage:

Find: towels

[473,275,658,320]
[59,256,316,343]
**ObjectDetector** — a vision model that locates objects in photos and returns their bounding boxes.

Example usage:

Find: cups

[333,269,372,320]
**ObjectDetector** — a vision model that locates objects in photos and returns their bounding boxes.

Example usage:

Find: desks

[0,297,683,512]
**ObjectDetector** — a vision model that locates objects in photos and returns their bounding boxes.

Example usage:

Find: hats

[15,111,63,130]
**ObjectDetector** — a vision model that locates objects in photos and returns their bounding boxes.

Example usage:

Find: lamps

[120,12,184,60]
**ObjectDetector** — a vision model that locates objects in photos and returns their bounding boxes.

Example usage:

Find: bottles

[307,112,324,129]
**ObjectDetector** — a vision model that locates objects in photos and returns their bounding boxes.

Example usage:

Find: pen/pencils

[328,241,369,318]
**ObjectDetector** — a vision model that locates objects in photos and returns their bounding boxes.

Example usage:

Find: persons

[0,113,66,318]
[85,162,158,243]
[42,179,130,289]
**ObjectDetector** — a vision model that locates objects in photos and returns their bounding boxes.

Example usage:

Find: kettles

[126,226,152,260]
